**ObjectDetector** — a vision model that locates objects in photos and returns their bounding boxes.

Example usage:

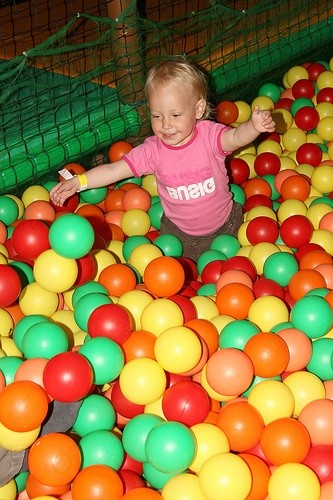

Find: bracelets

[74,173,89,193]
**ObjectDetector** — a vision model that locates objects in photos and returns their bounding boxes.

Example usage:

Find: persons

[48,57,277,263]
